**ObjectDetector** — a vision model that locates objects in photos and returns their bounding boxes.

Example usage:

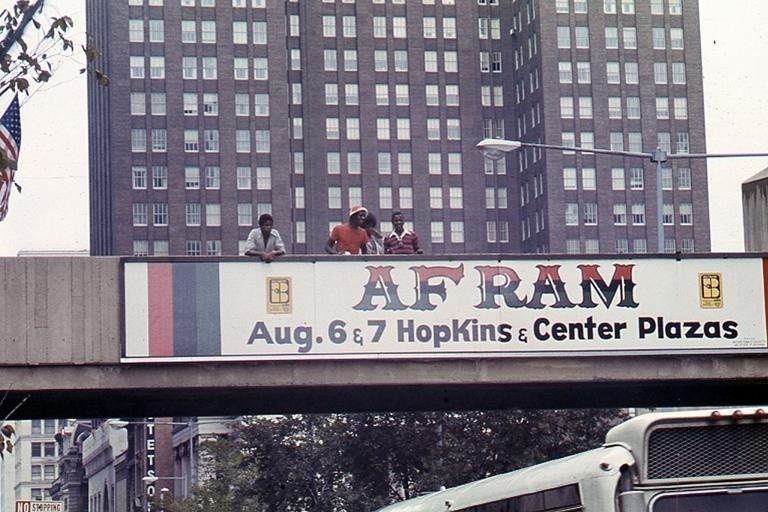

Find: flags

[0,93,22,221]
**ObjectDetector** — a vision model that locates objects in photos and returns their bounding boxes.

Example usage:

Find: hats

[349,205,368,218]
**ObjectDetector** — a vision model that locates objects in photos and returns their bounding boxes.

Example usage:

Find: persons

[325,206,369,254]
[384,212,424,254]
[360,213,384,254]
[243,213,287,263]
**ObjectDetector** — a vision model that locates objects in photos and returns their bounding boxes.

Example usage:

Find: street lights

[473,138,767,254]
[110,420,194,511]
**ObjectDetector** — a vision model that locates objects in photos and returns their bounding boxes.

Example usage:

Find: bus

[375,408,768,512]
